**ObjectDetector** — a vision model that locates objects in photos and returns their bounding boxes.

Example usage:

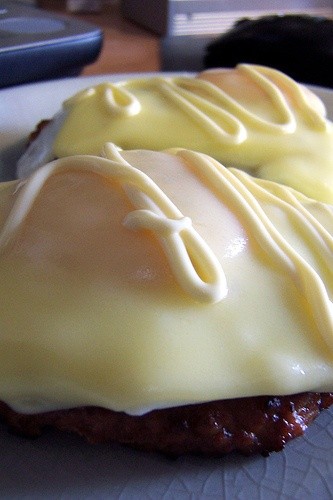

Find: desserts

[0,65,333,461]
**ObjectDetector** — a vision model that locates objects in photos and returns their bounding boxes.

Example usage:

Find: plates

[0,72,333,500]
